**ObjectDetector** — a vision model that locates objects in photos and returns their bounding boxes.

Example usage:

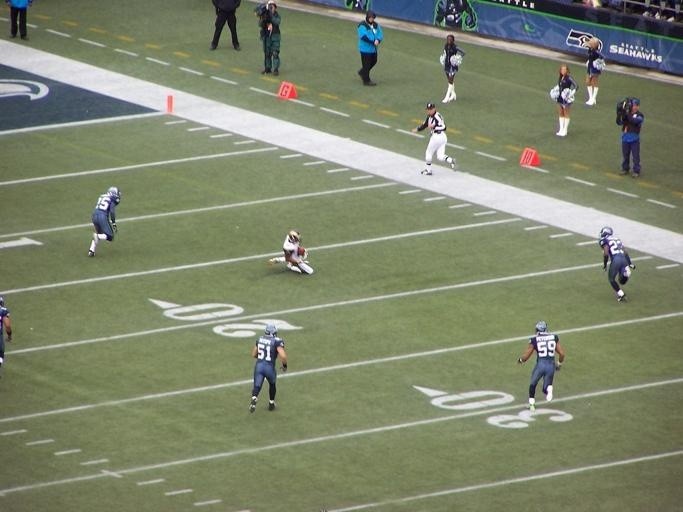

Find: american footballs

[298,247,305,256]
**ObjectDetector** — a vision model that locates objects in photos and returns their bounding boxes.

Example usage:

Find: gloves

[113,224,117,232]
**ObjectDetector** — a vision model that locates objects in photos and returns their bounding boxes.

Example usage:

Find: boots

[442,83,456,103]
[556,117,570,136]
[586,86,599,105]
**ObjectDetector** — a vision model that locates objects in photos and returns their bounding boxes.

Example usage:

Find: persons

[437,35,464,103]
[268,232,314,278]
[0,298,11,364]
[208,1,239,52]
[546,64,578,137]
[600,223,634,302]
[517,321,564,410]
[582,35,606,108]
[249,323,287,415]
[616,98,644,177]
[255,3,281,75]
[4,1,32,41]
[355,9,383,88]
[86,185,120,259]
[411,101,455,177]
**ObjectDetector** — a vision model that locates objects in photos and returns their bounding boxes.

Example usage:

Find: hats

[426,104,435,109]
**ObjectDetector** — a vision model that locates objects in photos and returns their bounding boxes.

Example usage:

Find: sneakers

[530,385,553,410]
[421,170,432,175]
[618,293,626,304]
[88,233,99,257]
[620,170,638,177]
[248,397,275,412]
[450,157,456,170]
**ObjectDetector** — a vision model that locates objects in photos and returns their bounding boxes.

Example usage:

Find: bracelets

[280,363,285,368]
[555,361,562,365]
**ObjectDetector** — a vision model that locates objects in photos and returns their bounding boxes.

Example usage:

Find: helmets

[288,231,300,243]
[536,321,547,332]
[601,227,613,237]
[265,324,277,337]
[107,186,120,200]
